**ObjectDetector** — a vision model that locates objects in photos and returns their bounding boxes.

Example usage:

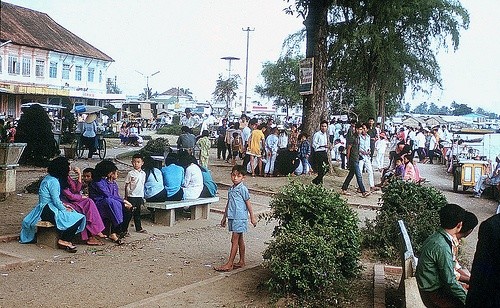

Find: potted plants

[59,100,80,159]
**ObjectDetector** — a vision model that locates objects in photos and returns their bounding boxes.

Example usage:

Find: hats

[85,114,96,124]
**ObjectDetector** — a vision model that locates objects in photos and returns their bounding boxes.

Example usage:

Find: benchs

[144,196,220,227]
[403,277,428,308]
[396,220,419,308]
[23,219,60,250]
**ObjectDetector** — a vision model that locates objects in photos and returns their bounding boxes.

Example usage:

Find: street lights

[220,56,241,108]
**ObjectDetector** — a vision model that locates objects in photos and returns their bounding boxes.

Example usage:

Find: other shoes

[136,230,146,233]
[370,187,379,192]
[363,192,370,196]
[356,188,362,192]
[341,191,350,196]
[183,207,191,213]
[311,181,316,186]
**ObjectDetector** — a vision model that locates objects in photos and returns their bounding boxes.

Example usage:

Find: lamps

[192,144,201,160]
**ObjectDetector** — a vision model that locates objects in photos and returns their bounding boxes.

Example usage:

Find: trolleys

[75,132,106,159]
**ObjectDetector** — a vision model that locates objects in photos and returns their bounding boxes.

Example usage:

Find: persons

[196,130,211,167]
[179,108,218,134]
[39,157,132,249]
[415,204,479,308]
[213,166,257,272]
[311,120,330,186]
[168,117,171,126]
[143,150,218,213]
[465,185,500,308]
[0,119,7,143]
[124,154,148,237]
[330,116,449,198]
[178,126,198,151]
[163,116,166,122]
[468,154,500,199]
[217,114,310,176]
[82,114,97,161]
[121,121,144,147]
